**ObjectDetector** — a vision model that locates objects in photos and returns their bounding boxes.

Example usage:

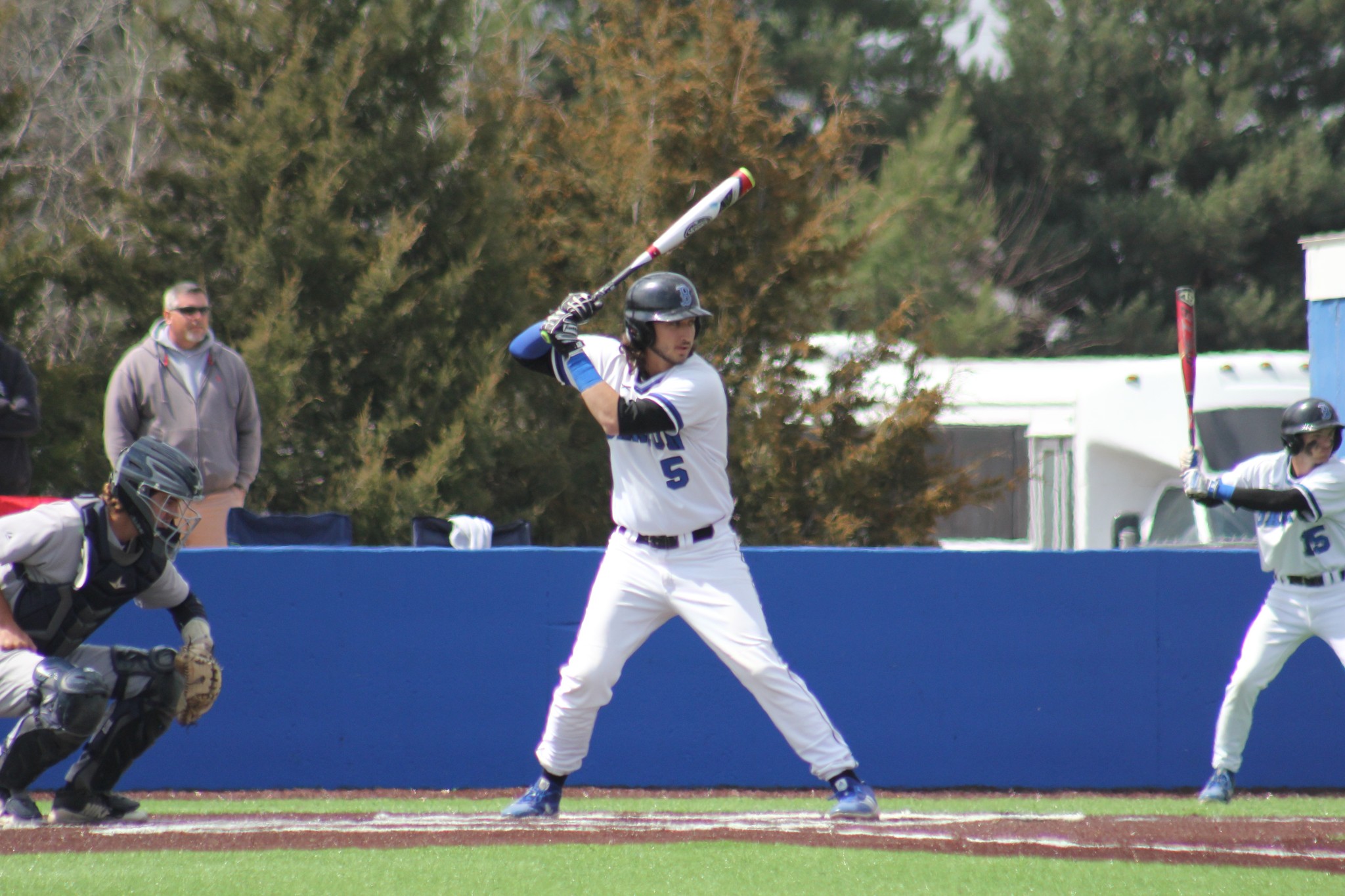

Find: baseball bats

[1172,283,1204,501]
[539,165,757,346]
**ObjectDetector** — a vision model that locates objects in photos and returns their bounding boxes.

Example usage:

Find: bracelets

[233,482,248,494]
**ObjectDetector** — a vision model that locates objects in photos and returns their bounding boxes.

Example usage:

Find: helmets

[1280,399,1343,456]
[108,434,204,565]
[624,272,713,348]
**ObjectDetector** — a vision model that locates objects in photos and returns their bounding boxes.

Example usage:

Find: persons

[499,272,880,819]
[0,337,41,496]
[103,281,263,550]
[1176,397,1345,804]
[0,433,222,826]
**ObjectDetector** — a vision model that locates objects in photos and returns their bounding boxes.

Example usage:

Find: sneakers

[827,776,880,819]
[500,774,562,817]
[0,787,44,831]
[47,784,140,823]
[1198,768,1233,801]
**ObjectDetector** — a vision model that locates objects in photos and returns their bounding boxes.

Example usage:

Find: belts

[1274,570,1345,588]
[614,524,714,549]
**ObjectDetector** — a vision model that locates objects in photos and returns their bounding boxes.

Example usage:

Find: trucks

[753,336,1312,548]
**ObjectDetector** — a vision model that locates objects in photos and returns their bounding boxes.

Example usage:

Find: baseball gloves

[175,633,223,732]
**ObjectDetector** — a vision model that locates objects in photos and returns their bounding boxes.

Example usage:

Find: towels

[447,514,494,550]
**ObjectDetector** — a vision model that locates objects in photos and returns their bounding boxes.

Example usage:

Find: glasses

[170,306,211,315]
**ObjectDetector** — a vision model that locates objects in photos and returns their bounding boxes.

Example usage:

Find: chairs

[227,509,359,547]
[411,513,530,551]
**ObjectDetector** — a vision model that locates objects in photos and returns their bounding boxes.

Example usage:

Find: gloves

[559,292,603,323]
[540,310,585,354]
[1179,467,1218,500]
[1180,445,1204,470]
